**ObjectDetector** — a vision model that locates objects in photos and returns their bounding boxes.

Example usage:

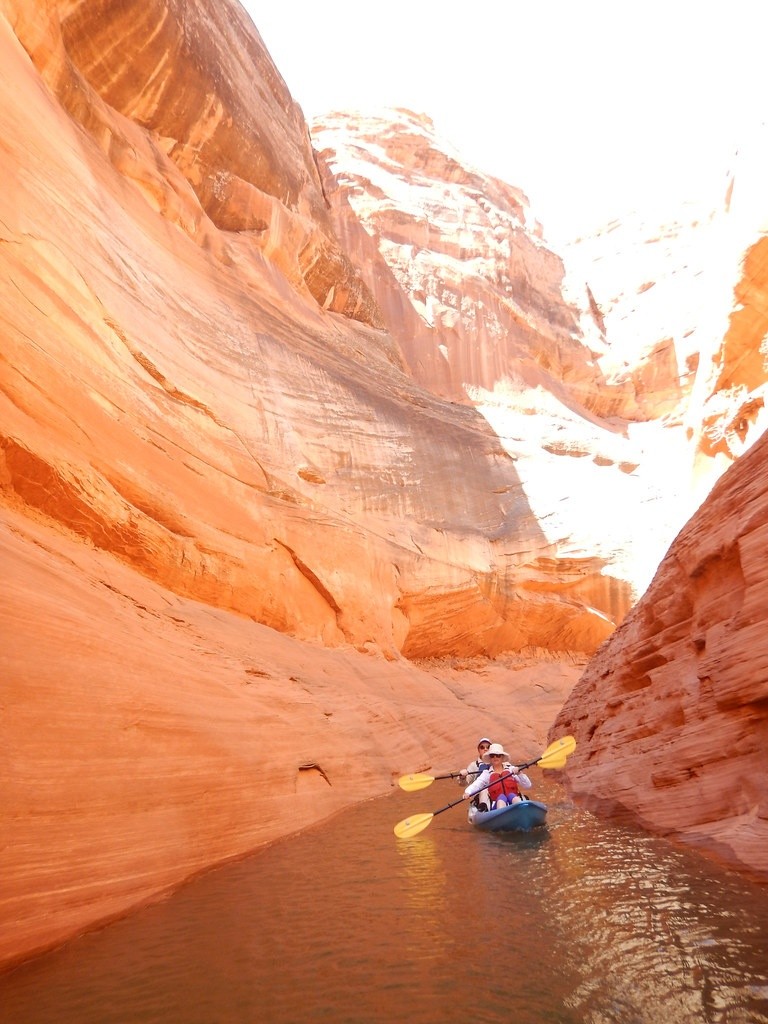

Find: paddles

[398,755,568,793]
[392,735,577,839]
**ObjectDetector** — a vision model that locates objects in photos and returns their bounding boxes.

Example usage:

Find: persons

[462,743,532,811]
[457,737,513,788]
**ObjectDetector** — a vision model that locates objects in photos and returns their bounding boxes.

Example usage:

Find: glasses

[489,754,501,758]
[480,745,490,749]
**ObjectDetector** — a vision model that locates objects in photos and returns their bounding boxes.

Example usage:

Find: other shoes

[478,803,487,813]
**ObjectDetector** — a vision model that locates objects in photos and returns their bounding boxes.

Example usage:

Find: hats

[482,743,510,763]
[477,738,492,752]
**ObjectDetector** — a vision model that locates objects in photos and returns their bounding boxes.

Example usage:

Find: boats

[466,800,548,836]
